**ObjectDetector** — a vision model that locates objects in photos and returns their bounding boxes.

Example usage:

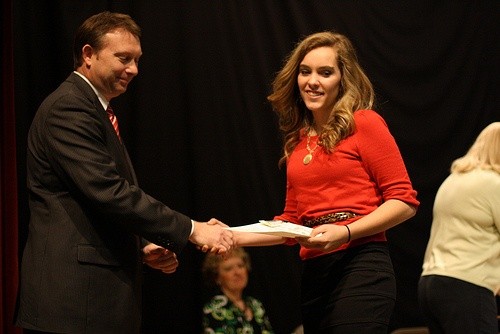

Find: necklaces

[301,127,319,164]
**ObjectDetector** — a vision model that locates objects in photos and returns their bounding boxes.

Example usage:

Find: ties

[106,105,122,144]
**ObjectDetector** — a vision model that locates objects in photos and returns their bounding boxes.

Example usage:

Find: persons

[420,121,500,334]
[14,10,232,333]
[197,31,420,334]
[201,246,278,334]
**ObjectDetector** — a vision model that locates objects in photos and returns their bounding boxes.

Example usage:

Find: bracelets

[345,225,352,243]
